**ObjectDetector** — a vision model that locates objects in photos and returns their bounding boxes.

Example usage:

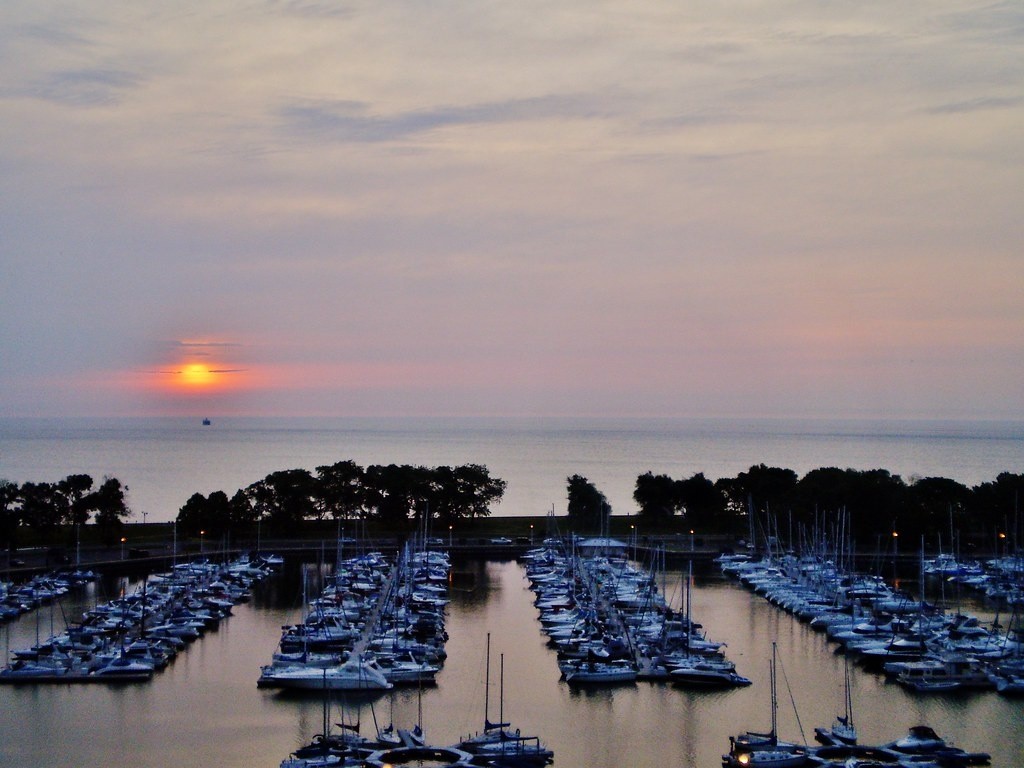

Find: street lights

[1000,533,1005,556]
[449,526,452,547]
[200,531,205,552]
[530,525,534,545]
[893,531,898,554]
[690,530,694,551]
[631,525,634,535]
[121,538,124,559]
[361,515,366,547]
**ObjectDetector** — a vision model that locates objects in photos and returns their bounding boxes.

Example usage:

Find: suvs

[423,536,444,546]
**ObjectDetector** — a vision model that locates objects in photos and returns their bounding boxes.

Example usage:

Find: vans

[516,536,534,544]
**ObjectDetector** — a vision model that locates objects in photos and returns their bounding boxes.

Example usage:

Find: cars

[337,536,357,545]
[736,539,744,547]
[746,542,753,550]
[542,538,563,545]
[565,535,580,543]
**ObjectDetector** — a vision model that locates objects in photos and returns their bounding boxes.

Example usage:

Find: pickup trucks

[491,537,512,545]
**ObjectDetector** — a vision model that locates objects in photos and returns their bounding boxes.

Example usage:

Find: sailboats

[256,497,451,691]
[520,489,753,690]
[720,639,992,768]
[712,485,1024,695]
[279,631,555,768]
[0,552,286,684]
